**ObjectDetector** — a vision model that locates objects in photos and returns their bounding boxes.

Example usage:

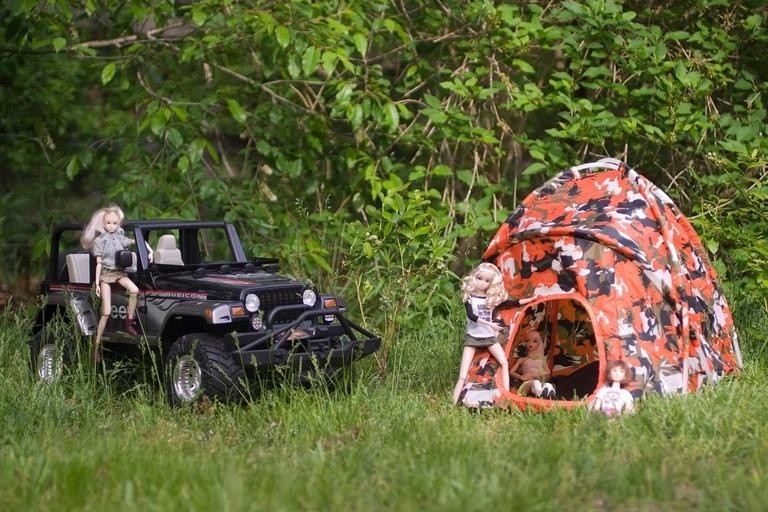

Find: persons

[510,328,557,400]
[28,218,381,414]
[81,205,154,364]
[451,264,538,403]
[591,359,635,417]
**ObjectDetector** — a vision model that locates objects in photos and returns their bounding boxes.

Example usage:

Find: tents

[457,156,744,415]
